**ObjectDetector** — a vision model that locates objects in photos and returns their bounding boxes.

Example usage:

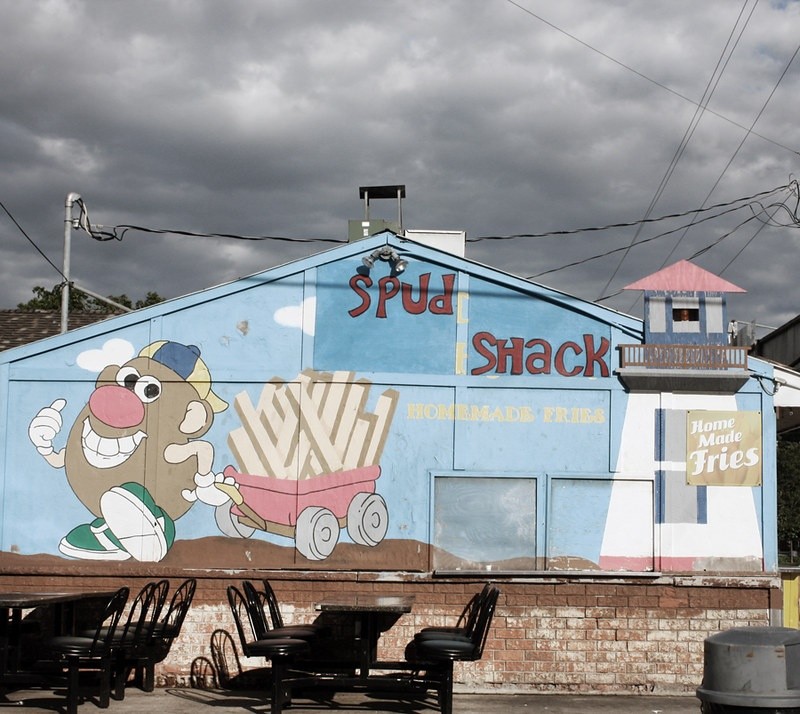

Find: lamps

[362,246,407,272]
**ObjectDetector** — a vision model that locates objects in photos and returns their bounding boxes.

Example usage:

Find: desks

[0,591,116,686]
[284,605,439,689]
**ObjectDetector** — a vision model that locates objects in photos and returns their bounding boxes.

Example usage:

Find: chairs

[42,577,197,714]
[414,581,500,714]
[226,578,323,714]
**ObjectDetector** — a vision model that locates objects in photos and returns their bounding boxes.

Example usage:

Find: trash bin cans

[696,626,800,714]
